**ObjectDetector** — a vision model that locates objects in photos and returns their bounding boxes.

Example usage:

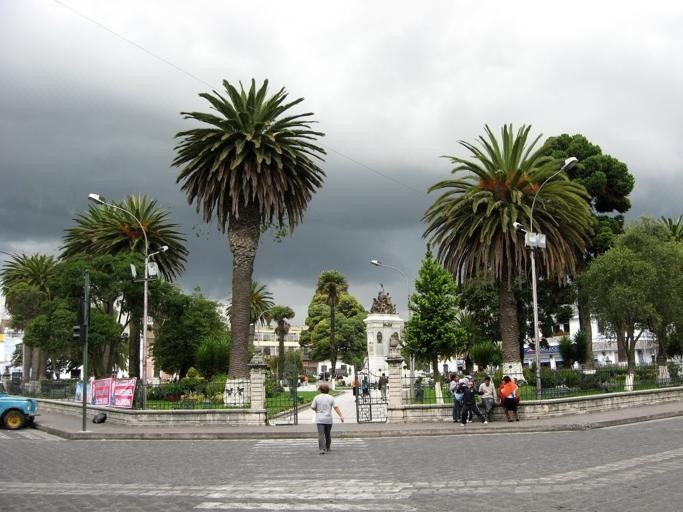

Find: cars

[1,383,42,430]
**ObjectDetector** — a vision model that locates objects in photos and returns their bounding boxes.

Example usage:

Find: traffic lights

[72,325,80,340]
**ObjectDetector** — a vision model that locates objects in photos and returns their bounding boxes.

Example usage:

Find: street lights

[512,156,579,400]
[369,259,416,401]
[86,193,170,409]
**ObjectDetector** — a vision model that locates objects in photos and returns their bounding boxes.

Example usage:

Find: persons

[302,374,309,387]
[361,375,369,402]
[109,381,116,406]
[310,383,344,455]
[75,384,80,401]
[90,382,96,404]
[296,376,301,387]
[351,376,360,401]
[413,370,520,427]
[377,373,389,401]
